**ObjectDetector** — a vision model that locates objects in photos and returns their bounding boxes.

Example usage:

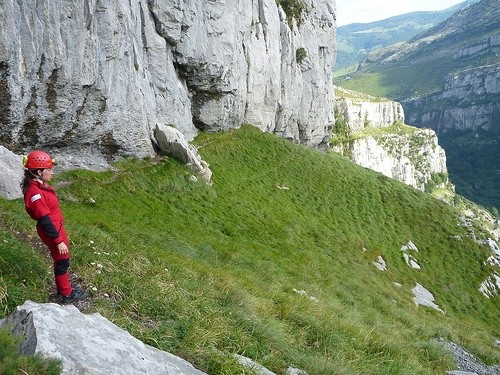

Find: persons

[20,150,92,300]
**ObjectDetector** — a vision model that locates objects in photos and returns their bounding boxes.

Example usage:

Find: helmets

[22,151,57,169]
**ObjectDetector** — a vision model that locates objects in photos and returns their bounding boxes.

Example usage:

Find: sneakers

[58,287,88,304]
